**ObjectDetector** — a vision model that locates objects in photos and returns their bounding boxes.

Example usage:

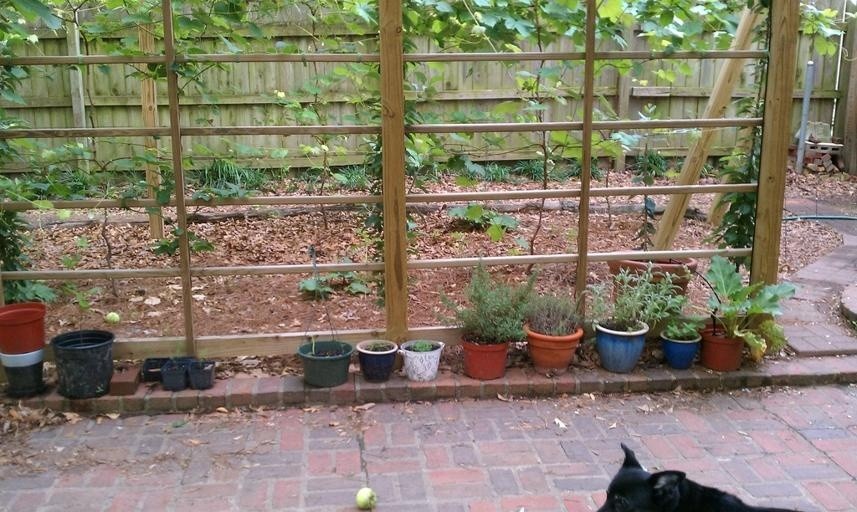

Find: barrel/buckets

[0,302,47,354]
[0,348,44,398]
[51,328,115,400]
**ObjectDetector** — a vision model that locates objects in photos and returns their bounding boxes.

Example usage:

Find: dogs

[596,441,802,512]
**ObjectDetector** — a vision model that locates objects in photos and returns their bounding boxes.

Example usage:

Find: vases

[297,338,399,388]
[0,303,216,400]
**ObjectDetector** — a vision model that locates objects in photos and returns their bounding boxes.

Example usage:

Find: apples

[356,487,377,509]
[105,312,121,323]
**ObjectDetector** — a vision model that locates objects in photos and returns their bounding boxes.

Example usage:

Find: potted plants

[660,315,707,370]
[691,254,797,373]
[523,287,584,377]
[584,259,684,373]
[399,339,446,383]
[434,263,539,381]
